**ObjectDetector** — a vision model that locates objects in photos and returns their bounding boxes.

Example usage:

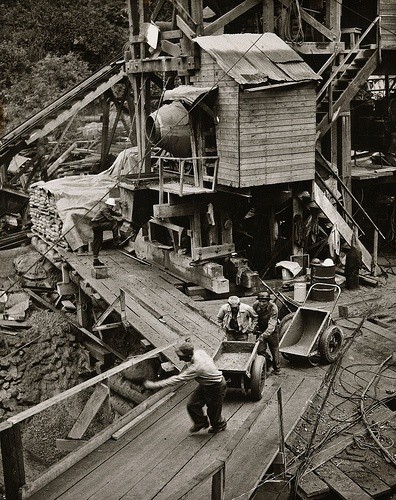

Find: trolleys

[211,329,267,401]
[277,283,345,367]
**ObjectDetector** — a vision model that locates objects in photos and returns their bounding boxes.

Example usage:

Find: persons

[143,342,227,433]
[217,296,259,341]
[91,198,123,266]
[253,291,281,374]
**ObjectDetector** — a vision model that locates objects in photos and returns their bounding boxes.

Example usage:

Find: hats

[175,341,195,358]
[227,295,241,308]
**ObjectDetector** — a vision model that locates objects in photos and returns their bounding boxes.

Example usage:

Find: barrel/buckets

[294,283,306,302]
[310,260,336,302]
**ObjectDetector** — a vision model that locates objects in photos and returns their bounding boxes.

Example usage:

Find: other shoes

[209,423,227,433]
[92,261,104,266]
[114,240,125,245]
[190,421,209,432]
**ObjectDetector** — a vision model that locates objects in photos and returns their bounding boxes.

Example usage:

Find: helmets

[255,291,272,302]
[105,198,117,207]
